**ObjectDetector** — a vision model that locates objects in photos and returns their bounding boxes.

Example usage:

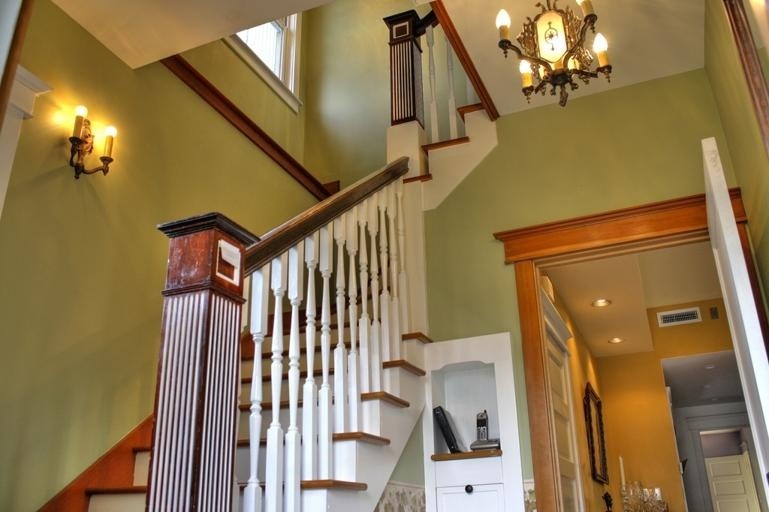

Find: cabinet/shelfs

[423,331,525,512]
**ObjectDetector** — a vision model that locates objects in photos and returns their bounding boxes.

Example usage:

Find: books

[434,405,469,454]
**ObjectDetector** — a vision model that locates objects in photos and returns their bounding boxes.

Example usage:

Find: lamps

[495,0,612,107]
[69,105,115,179]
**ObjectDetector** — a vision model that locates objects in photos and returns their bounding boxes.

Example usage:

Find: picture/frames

[583,381,610,485]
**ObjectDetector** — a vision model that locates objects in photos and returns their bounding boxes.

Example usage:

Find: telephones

[470,409,500,450]
